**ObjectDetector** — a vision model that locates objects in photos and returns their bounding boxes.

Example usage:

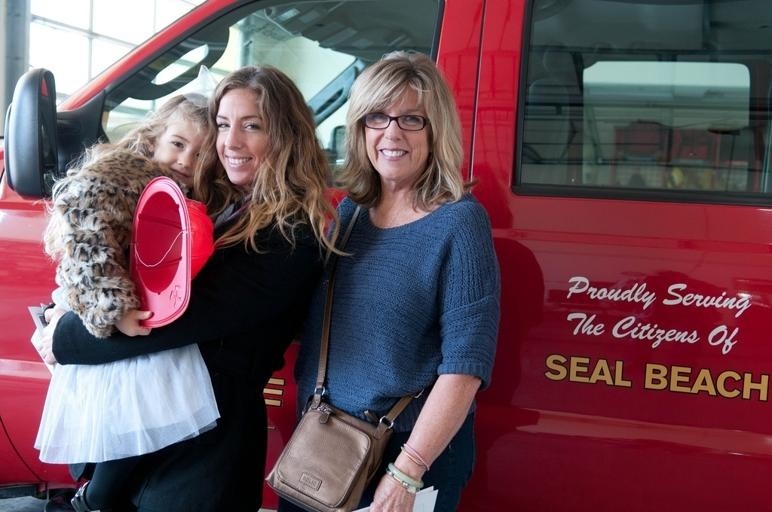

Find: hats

[124,170,222,332]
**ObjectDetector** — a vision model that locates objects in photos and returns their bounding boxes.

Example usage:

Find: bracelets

[384,443,430,494]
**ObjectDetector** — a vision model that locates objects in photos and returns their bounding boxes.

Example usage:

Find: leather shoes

[68,478,107,511]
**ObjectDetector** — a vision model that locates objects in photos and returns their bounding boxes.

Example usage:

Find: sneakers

[265,391,396,512]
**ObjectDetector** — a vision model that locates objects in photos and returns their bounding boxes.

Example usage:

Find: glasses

[358,108,434,134]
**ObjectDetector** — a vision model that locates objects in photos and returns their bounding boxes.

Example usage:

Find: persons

[32,65,338,512]
[277,48,502,512]
[41,92,221,512]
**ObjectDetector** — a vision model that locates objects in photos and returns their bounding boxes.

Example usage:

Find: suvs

[581,82,751,194]
[0,2,771,511]
